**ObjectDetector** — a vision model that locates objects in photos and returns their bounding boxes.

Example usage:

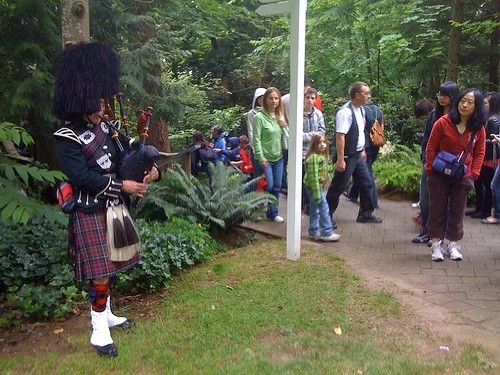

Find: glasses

[305,96,315,99]
[436,93,449,98]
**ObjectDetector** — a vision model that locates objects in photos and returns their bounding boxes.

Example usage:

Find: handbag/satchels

[192,161,202,175]
[370,106,386,146]
[282,128,289,150]
[432,151,463,180]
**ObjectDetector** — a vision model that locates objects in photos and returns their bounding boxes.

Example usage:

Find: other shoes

[333,224,337,228]
[91,319,136,328]
[465,211,478,215]
[375,205,379,208]
[357,216,383,223]
[412,203,421,208]
[471,212,491,218]
[344,192,358,202]
[481,216,500,224]
[94,341,118,356]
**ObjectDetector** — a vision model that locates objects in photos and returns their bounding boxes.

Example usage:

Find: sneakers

[320,232,340,241]
[308,231,320,240]
[427,239,444,246]
[255,216,262,222]
[447,243,463,260]
[267,215,284,223]
[430,240,444,261]
[412,233,430,243]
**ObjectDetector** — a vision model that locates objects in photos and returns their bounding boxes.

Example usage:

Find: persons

[52,83,161,358]
[347,88,383,208]
[190,88,268,222]
[411,99,435,209]
[411,79,460,247]
[282,74,326,217]
[302,133,346,242]
[253,87,288,223]
[464,92,500,224]
[327,81,384,229]
[423,88,486,261]
